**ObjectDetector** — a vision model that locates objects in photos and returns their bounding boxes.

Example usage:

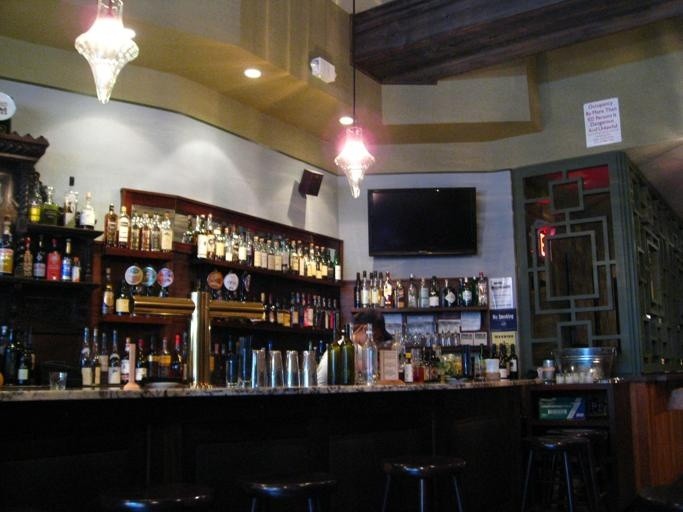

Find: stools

[249,474,337,511]
[522,431,601,511]
[384,457,468,511]
[122,485,211,511]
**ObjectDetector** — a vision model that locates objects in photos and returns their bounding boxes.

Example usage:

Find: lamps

[332,0,376,200]
[74,0,143,109]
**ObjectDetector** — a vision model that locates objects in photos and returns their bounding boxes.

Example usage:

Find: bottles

[358,324,378,386]
[98,259,171,320]
[207,337,324,387]
[78,325,190,387]
[182,210,344,282]
[352,270,488,308]
[28,173,173,254]
[0,317,35,387]
[202,267,340,333]
[325,326,355,386]
[477,341,519,379]
[0,220,82,288]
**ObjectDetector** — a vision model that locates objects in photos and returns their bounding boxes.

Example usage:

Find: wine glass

[383,319,472,384]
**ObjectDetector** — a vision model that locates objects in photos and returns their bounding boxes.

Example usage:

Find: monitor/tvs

[368,187,477,256]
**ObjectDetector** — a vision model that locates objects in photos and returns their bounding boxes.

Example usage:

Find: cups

[49,371,67,390]
[542,359,556,381]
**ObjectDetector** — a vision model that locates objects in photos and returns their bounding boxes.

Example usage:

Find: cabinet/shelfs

[341,276,490,382]
[103,189,341,381]
[3,173,100,384]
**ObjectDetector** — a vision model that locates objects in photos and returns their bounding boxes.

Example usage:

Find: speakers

[298,169,324,196]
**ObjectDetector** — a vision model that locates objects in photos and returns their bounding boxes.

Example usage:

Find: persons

[316,308,391,384]
